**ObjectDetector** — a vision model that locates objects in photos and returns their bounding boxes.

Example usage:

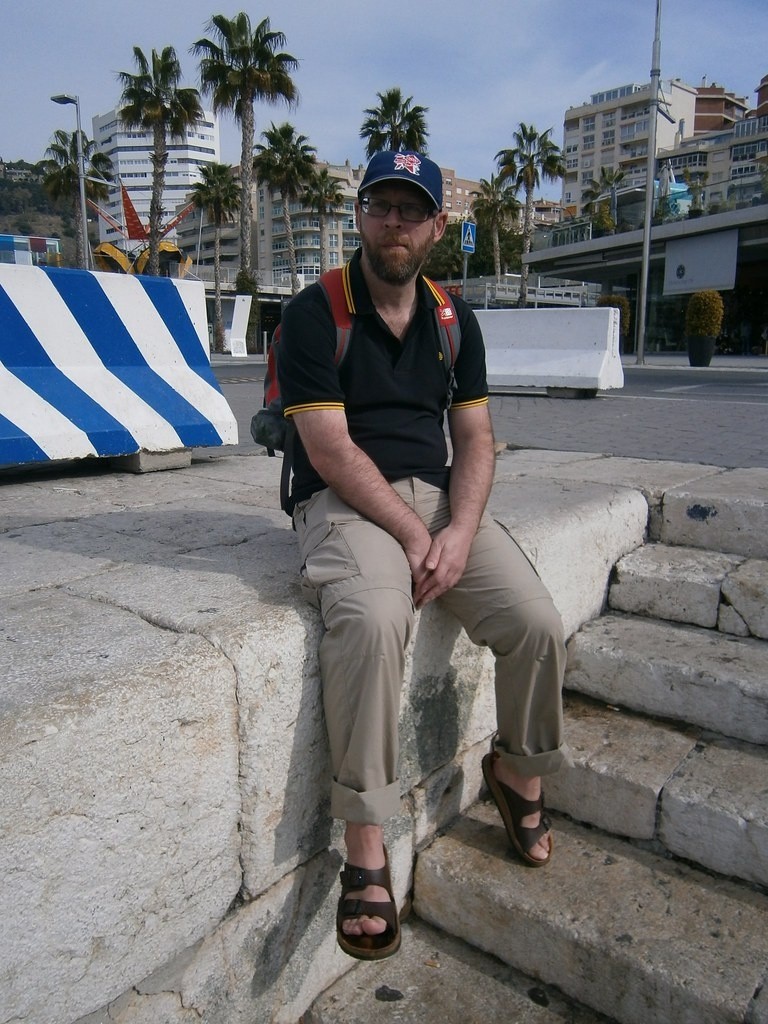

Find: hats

[355,148,444,216]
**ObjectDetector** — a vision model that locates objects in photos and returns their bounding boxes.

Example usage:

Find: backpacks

[247,262,460,457]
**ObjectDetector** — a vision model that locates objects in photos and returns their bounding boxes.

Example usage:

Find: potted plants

[595,295,631,355]
[683,288,725,368]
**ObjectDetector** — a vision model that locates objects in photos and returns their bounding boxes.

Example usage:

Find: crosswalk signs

[461,220,477,255]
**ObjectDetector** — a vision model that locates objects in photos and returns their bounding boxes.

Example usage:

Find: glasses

[356,196,433,223]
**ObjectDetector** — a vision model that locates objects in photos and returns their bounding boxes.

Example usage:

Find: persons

[275,151,571,959]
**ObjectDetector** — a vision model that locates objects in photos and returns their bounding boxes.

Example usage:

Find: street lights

[50,94,89,271]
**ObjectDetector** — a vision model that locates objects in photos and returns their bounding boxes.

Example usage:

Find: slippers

[480,750,555,865]
[334,842,402,960]
